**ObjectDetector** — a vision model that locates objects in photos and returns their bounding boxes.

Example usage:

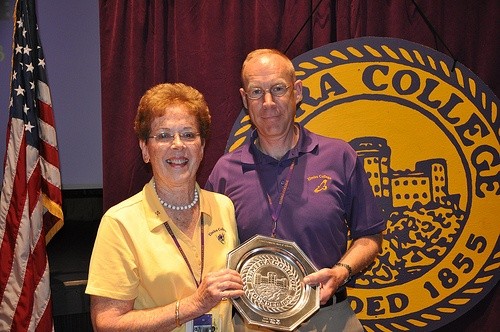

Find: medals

[227,234,320,331]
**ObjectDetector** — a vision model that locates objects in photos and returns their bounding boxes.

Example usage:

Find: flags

[0,0,64,332]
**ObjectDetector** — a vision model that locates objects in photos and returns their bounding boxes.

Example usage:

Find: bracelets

[331,263,352,287]
[176,299,183,328]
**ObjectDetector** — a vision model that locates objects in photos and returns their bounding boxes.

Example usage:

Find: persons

[85,48,387,332]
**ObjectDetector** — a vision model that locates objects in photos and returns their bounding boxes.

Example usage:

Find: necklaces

[153,181,198,210]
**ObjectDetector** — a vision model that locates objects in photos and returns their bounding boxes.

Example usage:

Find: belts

[320,287,348,308]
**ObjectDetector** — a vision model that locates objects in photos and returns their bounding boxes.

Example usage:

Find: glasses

[243,83,294,99]
[145,130,202,141]
